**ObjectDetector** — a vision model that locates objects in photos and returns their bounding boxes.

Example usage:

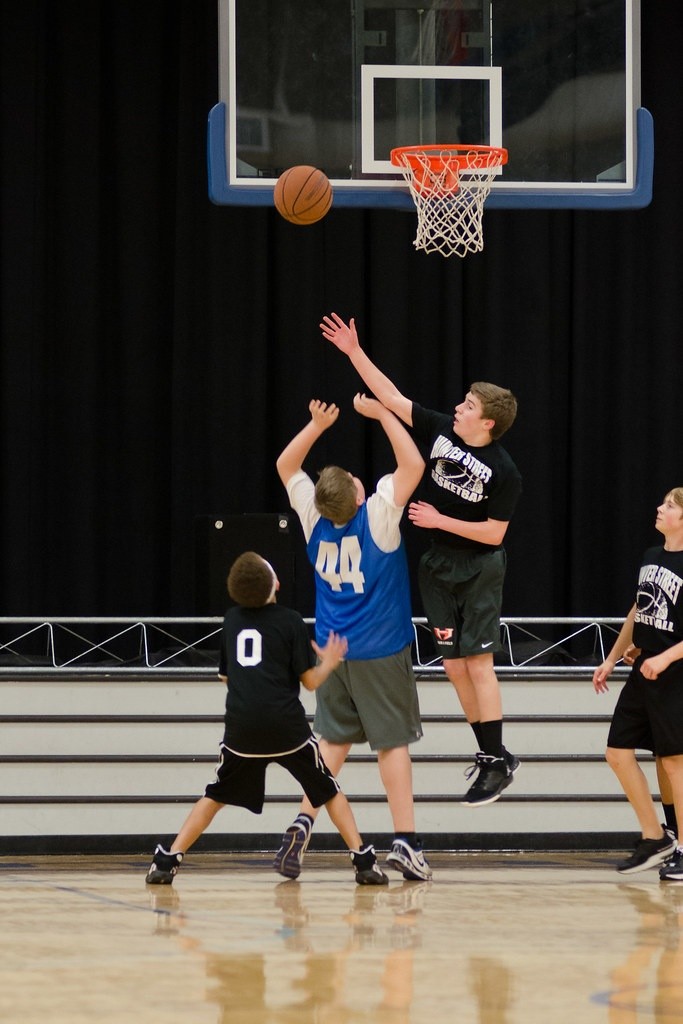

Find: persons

[593,486,683,882]
[319,313,523,806]
[144,550,388,886]
[272,394,431,881]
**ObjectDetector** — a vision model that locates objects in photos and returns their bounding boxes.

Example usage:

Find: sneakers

[617,823,676,874]
[385,838,433,881]
[273,818,312,879]
[348,843,389,884]
[146,844,184,885]
[659,848,683,880]
[460,744,521,807]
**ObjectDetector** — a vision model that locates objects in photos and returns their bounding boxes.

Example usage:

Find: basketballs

[274,164,334,226]
